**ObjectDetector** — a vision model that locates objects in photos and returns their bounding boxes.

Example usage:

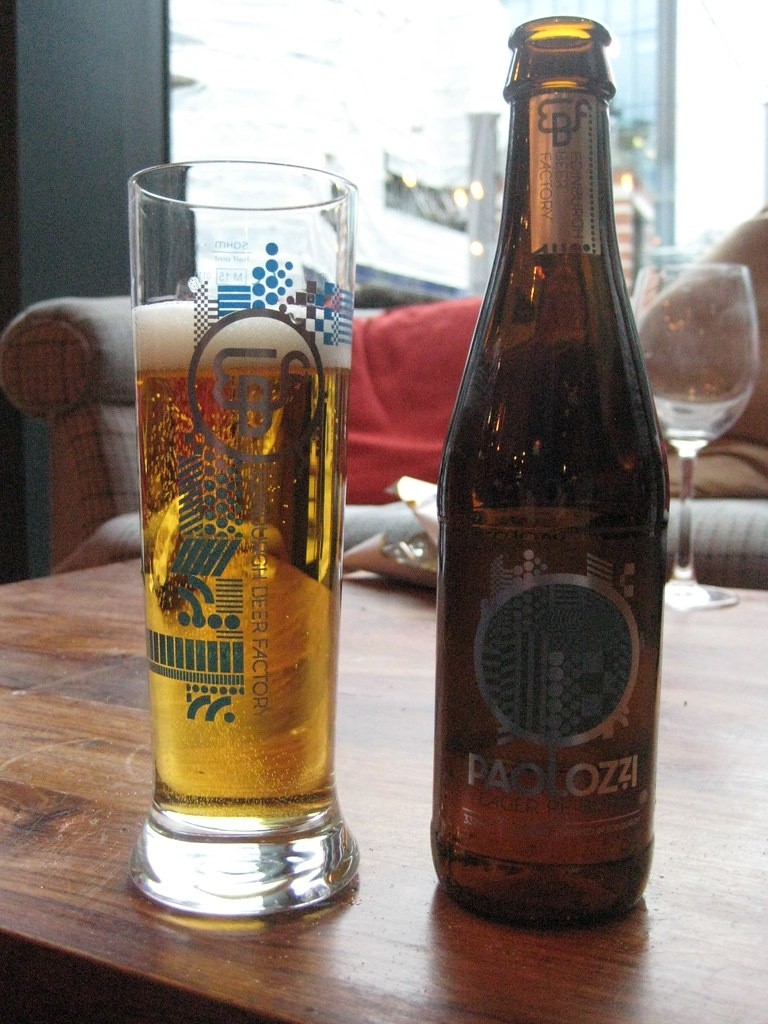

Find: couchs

[0,295,768,592]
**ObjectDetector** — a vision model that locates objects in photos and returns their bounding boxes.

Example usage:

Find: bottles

[428,13,668,928]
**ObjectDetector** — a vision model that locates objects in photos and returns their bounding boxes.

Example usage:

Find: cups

[125,158,362,920]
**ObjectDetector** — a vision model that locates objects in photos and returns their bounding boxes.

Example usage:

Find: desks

[0,548,768,1024]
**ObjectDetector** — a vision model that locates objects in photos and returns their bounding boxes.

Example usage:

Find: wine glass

[624,262,760,612]
